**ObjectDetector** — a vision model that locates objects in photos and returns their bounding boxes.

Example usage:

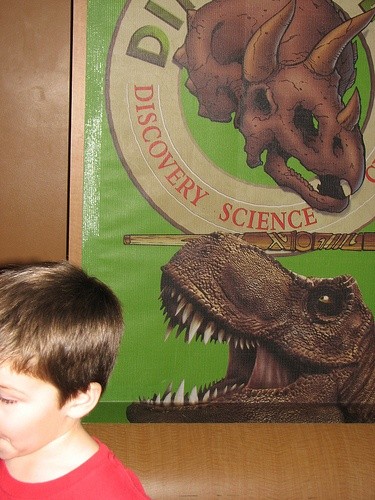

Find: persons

[1,259,154,500]
